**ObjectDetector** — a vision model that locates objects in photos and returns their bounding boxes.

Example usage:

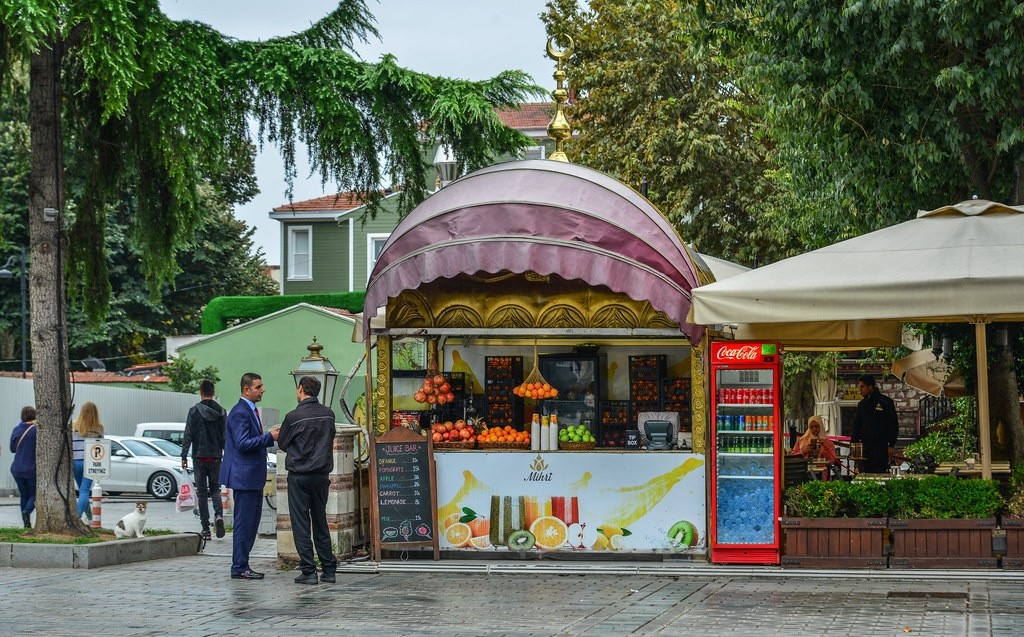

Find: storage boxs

[438,354,692,447]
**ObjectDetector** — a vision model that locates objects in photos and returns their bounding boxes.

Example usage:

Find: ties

[255,408,263,434]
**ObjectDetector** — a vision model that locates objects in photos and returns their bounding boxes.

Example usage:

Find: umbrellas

[685,196,1023,479]
[890,345,973,461]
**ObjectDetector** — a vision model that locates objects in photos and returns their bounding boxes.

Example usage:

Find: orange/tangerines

[477,425,530,443]
[487,357,513,423]
[601,357,690,434]
[529,516,569,549]
[444,514,492,549]
[513,382,559,399]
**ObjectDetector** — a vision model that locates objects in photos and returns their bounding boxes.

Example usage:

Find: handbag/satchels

[176,468,195,511]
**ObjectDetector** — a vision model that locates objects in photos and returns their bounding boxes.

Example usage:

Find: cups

[530,414,558,450]
[488,494,579,547]
[891,466,899,476]
[966,459,975,470]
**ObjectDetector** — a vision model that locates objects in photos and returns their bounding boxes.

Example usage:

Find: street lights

[1,256,26,380]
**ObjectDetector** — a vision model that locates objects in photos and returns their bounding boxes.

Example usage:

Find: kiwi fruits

[508,530,536,551]
[668,520,699,553]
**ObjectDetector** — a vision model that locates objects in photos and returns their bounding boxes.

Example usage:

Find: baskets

[478,442,530,449]
[558,440,596,450]
[434,441,475,449]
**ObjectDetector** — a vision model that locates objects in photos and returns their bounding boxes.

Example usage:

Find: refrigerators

[711,341,782,564]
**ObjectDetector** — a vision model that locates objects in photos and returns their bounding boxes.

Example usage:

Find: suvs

[134,423,277,474]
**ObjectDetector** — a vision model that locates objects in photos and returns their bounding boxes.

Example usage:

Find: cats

[113,501,149,539]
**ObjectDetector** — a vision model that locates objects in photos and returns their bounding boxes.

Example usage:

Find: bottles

[718,436,774,543]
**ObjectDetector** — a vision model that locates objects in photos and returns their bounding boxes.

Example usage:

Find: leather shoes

[231,568,264,579]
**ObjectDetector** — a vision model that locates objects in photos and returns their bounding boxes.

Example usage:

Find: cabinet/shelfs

[537,352,608,447]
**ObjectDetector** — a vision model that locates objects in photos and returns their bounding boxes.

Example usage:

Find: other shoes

[24,524,31,529]
[22,510,30,525]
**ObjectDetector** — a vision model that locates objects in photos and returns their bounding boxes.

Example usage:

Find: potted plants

[776,476,1024,571]
[905,430,959,474]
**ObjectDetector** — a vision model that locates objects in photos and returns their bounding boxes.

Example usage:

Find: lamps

[288,336,340,410]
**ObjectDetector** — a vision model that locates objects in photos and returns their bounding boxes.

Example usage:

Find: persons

[72,402,104,525]
[181,380,227,540]
[218,373,280,579]
[278,376,337,584]
[850,374,898,474]
[10,406,37,528]
[789,416,835,482]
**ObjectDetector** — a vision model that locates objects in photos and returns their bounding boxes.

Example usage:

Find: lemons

[592,524,626,551]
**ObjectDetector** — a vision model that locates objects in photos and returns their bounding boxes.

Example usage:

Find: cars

[75,434,210,500]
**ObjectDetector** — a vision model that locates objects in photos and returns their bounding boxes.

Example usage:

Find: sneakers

[214,516,225,538]
[295,572,318,584]
[201,530,212,540]
[320,572,336,583]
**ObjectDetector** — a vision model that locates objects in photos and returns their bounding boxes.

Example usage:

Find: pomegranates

[414,375,454,405]
[430,419,478,445]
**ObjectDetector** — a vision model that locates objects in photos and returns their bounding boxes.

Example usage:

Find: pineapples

[470,417,487,435]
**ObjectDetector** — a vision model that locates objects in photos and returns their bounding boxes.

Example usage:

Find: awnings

[363,159,702,346]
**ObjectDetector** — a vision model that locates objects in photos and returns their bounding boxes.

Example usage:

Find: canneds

[717,387,773,404]
[715,415,774,431]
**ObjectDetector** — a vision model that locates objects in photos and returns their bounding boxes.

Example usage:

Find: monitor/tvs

[637,412,679,451]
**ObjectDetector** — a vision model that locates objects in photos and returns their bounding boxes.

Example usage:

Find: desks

[836,454,850,475]
[809,458,834,481]
[890,459,1011,474]
[807,464,829,480]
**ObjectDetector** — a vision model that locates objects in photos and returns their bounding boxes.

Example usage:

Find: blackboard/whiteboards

[369,427,439,547]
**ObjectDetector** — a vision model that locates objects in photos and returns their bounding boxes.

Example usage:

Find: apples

[566,522,598,548]
[558,424,596,443]
